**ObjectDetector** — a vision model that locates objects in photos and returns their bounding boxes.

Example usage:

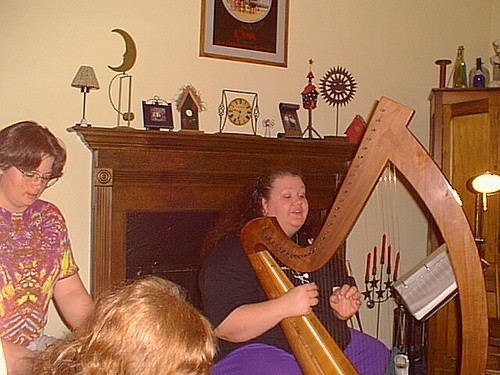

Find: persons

[30,275,218,375]
[198,165,390,375]
[0,121,94,375]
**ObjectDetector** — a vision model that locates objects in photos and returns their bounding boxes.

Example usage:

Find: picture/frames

[142,95,174,130]
[199,0,289,68]
[278,102,303,140]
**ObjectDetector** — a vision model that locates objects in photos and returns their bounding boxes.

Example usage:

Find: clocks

[217,89,259,135]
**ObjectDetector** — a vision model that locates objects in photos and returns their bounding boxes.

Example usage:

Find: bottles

[452,45,467,89]
[473,57,485,87]
[468,66,490,88]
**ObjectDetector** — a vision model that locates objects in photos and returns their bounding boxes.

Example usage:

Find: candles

[366,234,401,277]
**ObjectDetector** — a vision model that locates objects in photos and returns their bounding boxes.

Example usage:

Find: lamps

[71,66,100,128]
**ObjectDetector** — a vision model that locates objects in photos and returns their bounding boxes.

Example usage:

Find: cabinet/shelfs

[428,89,500,375]
[67,126,364,310]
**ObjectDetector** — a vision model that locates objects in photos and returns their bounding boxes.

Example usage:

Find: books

[392,243,489,323]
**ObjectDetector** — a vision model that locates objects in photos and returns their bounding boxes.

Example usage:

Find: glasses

[14,165,59,189]
